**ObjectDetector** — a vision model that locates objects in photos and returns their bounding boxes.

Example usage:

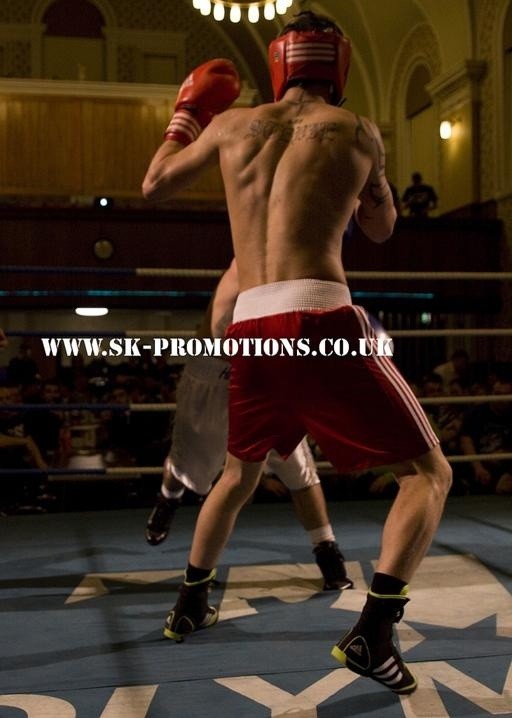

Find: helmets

[270,31,352,101]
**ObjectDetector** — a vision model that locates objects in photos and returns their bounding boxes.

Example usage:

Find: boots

[164,573,219,643]
[332,584,416,694]
[145,490,183,545]
[310,541,354,594]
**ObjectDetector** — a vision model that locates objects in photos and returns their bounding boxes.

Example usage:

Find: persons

[1,327,511,517]
[143,11,454,694]
[143,210,357,594]
[394,170,438,220]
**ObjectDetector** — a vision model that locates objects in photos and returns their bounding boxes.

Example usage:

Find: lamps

[190,0,291,24]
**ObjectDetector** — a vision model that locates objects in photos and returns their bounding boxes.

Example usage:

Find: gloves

[162,58,240,145]
